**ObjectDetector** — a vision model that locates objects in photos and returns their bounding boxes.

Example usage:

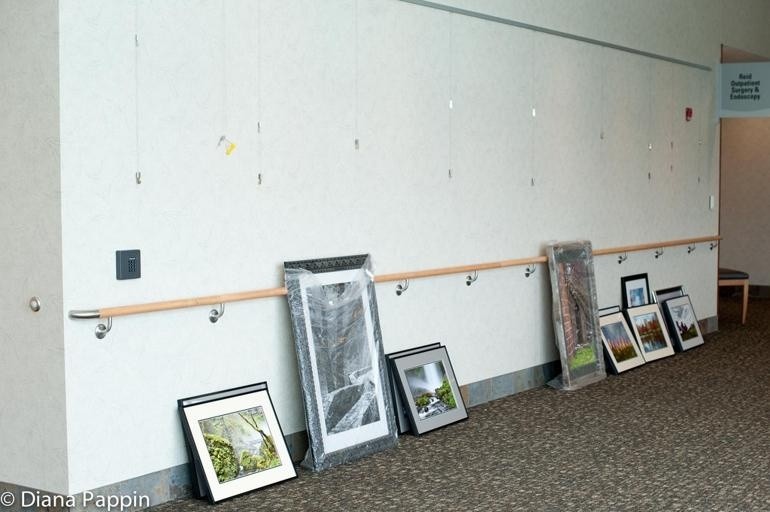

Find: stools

[718,268,749,326]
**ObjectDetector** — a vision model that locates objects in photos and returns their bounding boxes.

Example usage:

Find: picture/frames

[391,346,469,437]
[177,379,268,496]
[384,342,442,433]
[282,253,400,473]
[179,388,298,503]
[545,239,708,389]
